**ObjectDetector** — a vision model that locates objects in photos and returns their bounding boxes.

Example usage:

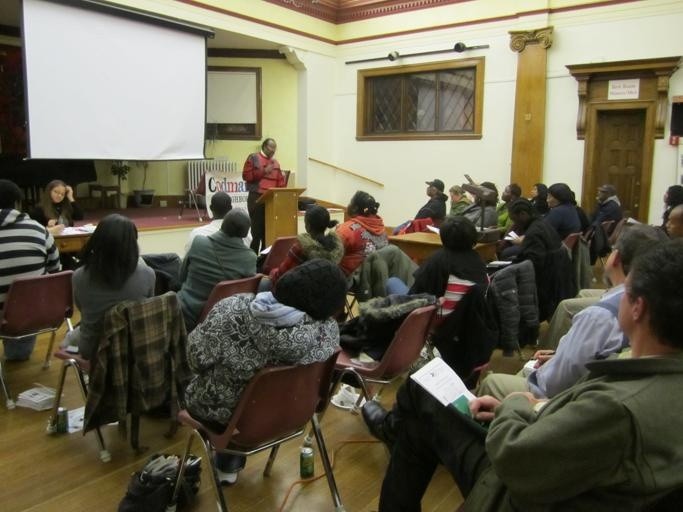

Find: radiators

[186,160,237,192]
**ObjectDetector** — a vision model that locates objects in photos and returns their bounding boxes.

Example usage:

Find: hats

[547,183,572,203]
[425,179,444,191]
[272,258,347,320]
[596,184,617,196]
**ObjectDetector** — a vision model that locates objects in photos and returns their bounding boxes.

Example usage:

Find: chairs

[303,296,446,446]
[344,245,409,322]
[427,272,495,389]
[166,344,344,512]
[544,230,584,321]
[0,270,73,410]
[259,236,299,275]
[588,220,614,265]
[139,252,183,296]
[46,291,183,463]
[487,260,542,362]
[608,215,632,246]
[198,273,263,326]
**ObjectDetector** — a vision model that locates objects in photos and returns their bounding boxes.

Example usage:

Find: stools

[88,184,120,211]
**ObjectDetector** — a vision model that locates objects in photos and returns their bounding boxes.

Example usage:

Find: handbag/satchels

[118,452,202,512]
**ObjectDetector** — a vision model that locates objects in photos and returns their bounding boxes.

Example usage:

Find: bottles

[56,407,68,432]
[300,448,314,477]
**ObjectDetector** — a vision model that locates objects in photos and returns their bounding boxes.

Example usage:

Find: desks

[388,232,524,266]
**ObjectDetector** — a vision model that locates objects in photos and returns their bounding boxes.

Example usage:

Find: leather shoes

[361,400,389,440]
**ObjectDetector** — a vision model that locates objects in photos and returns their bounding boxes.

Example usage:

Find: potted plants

[110,160,155,209]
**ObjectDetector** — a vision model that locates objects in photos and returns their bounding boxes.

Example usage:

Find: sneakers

[216,467,239,486]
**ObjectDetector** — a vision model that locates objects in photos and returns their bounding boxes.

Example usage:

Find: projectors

[460,173,498,207]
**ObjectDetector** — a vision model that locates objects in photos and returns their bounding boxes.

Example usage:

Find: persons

[589,185,621,236]
[392,179,447,236]
[184,192,235,256]
[570,189,589,236]
[470,223,673,403]
[537,288,609,349]
[448,185,473,218]
[661,185,683,231]
[495,183,522,238]
[185,259,345,488]
[241,137,287,257]
[259,205,345,293]
[175,208,258,333]
[461,182,499,229]
[32,179,85,271]
[665,205,683,236]
[335,190,390,275]
[0,178,64,361]
[526,184,546,209]
[59,212,157,352]
[498,197,563,273]
[384,216,487,296]
[544,182,579,238]
[360,238,682,512]
